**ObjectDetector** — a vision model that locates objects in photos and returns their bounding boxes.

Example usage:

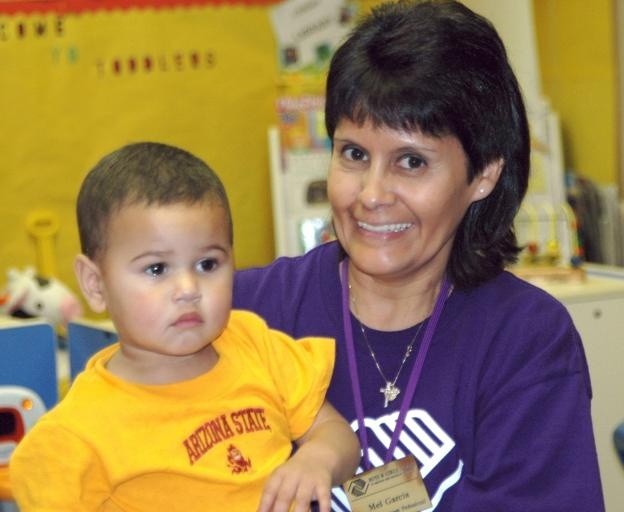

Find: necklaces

[347,281,433,410]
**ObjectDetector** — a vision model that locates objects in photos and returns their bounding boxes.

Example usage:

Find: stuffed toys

[1,263,81,339]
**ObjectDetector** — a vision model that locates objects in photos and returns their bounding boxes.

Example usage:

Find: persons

[6,140,363,512]
[227,1,607,512]
